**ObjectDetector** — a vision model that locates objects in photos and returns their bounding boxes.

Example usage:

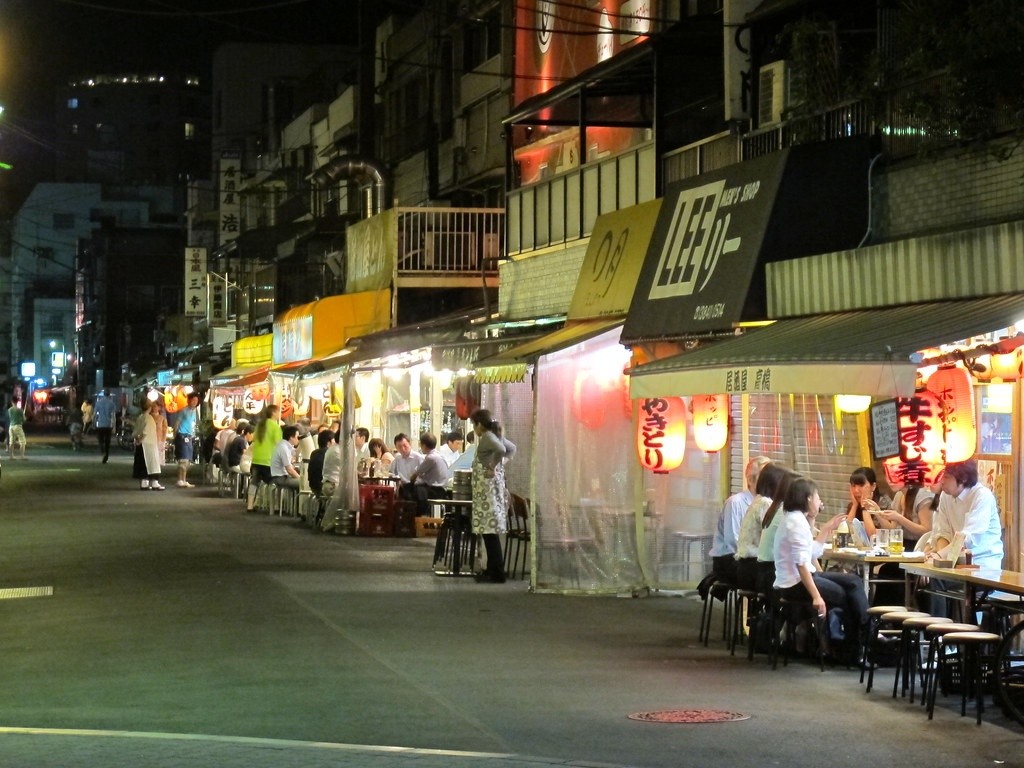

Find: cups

[819,499,823,513]
[871,529,889,550]
[890,529,903,553]
[832,530,837,550]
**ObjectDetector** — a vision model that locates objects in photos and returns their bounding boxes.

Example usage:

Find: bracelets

[937,552,942,559]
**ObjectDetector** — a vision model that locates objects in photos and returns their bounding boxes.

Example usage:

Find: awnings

[623,296,1024,397]
[431,317,627,384]
[205,288,472,387]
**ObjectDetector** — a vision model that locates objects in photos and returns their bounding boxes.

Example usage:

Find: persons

[203,404,477,532]
[132,392,199,490]
[844,461,1004,670]
[67,388,117,464]
[469,409,516,584]
[0,396,27,460]
[708,456,878,670]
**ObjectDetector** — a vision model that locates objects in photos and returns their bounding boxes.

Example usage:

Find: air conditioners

[756,60,788,126]
[424,230,476,268]
[484,233,499,259]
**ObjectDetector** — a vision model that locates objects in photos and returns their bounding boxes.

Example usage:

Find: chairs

[502,491,585,589]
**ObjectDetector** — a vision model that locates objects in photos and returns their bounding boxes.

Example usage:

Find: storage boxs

[352,484,443,538]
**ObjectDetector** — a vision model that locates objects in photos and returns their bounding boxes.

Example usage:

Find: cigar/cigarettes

[818,614,823,618]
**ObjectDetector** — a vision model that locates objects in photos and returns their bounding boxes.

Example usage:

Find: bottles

[956,546,966,566]
[836,518,849,548]
[369,463,374,477]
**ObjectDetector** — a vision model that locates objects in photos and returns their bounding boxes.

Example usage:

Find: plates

[867,510,884,515]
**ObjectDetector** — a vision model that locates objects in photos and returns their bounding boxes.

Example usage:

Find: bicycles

[991,619,1023,726]
[115,428,135,452]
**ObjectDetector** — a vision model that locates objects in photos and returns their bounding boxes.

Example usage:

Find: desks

[555,501,616,563]
[822,549,927,610]
[596,506,661,589]
[426,497,479,576]
[900,561,1024,702]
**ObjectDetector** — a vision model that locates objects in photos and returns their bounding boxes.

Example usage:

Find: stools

[209,460,339,534]
[670,532,1004,726]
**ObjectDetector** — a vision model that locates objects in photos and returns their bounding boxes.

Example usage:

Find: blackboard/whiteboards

[869,397,901,461]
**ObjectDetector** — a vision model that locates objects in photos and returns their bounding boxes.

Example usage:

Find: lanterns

[164,385,192,414]
[551,362,637,430]
[212,382,361,430]
[885,366,977,485]
[34,390,46,403]
[638,398,687,475]
[692,397,729,453]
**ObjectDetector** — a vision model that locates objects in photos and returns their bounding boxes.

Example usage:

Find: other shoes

[149,479,165,491]
[473,568,506,583]
[140,478,153,490]
[177,480,196,488]
[102,456,108,464]
[841,653,878,671]
